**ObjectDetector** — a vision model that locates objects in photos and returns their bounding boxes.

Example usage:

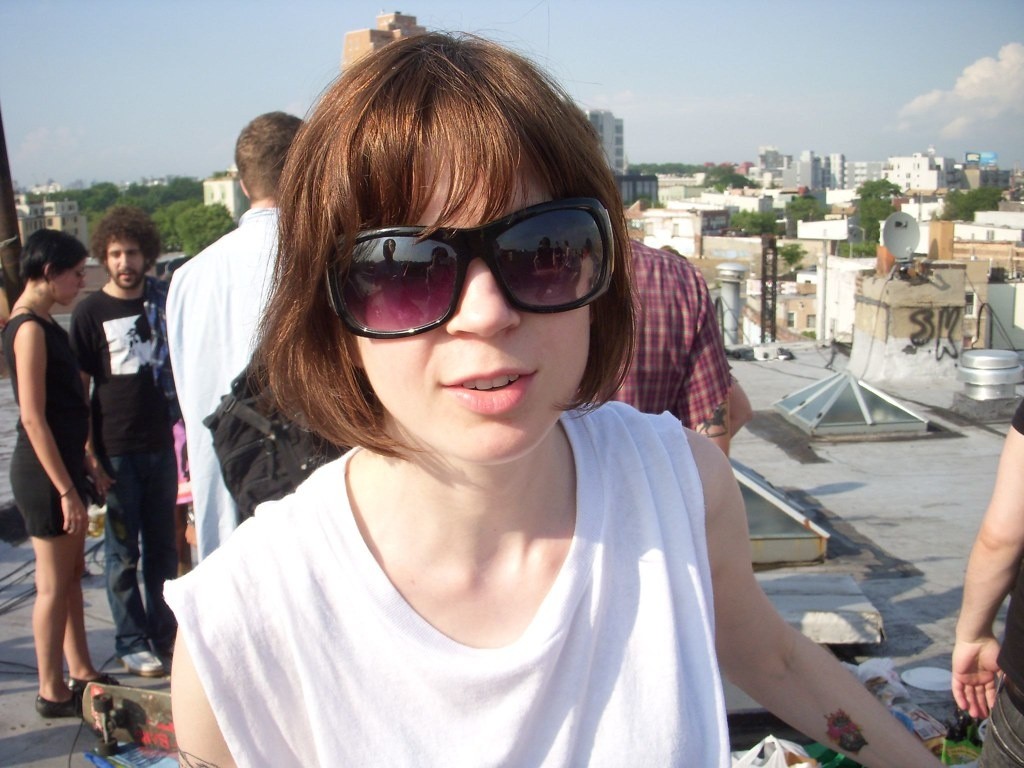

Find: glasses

[322,197,616,338]
[71,269,87,281]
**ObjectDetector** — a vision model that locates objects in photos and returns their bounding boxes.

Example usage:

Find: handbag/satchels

[731,734,822,768]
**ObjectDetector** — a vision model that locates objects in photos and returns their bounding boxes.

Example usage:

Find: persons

[165,112,307,566]
[369,237,600,323]
[70,206,193,678]
[952,397,1023,768]
[0,228,120,717]
[595,235,752,458]
[160,34,947,768]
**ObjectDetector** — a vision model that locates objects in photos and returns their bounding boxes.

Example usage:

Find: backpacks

[202,331,375,520]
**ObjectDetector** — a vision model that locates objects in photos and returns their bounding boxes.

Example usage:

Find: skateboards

[82,680,178,758]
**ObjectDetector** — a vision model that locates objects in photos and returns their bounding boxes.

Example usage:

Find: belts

[1003,672,1024,715]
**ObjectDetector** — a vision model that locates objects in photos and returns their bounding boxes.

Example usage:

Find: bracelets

[60,486,75,497]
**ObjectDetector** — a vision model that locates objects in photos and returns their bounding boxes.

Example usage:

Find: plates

[899,666,953,691]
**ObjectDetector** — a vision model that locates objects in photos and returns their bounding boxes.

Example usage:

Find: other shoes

[68,674,119,692]
[35,694,83,716]
[116,650,164,677]
